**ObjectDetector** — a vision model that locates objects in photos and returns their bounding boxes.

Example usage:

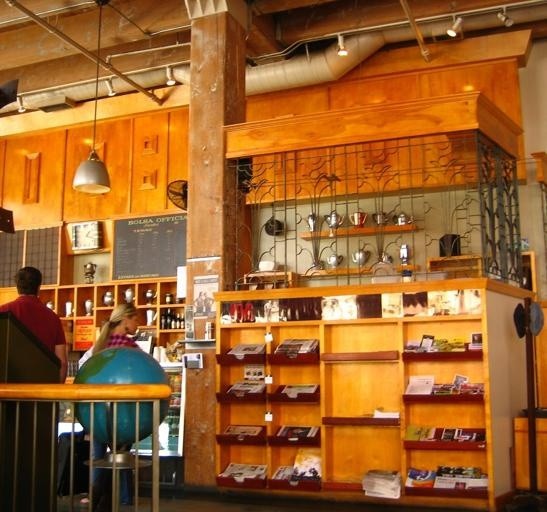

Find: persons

[0,267,67,384]
[91,302,143,506]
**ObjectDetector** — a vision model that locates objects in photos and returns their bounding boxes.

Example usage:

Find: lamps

[17,96,26,113]
[337,33,348,56]
[105,77,116,97]
[447,16,463,38]
[72,0,111,194]
[166,66,176,86]
[497,7,514,27]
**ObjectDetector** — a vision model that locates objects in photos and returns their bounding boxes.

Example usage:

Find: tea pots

[303,212,317,232]
[351,248,371,266]
[324,210,346,227]
[143,288,158,305]
[123,286,135,304]
[397,212,415,226]
[372,211,391,226]
[349,206,369,226]
[326,252,344,267]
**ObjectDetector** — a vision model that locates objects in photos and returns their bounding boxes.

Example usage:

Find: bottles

[165,293,173,304]
[160,308,185,329]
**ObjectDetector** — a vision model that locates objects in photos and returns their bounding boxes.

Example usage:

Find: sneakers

[80,498,89,504]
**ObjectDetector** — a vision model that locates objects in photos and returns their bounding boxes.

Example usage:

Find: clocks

[71,221,103,250]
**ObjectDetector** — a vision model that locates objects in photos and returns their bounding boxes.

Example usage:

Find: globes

[76,346,172,471]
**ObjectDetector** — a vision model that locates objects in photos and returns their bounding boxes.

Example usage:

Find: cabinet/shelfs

[40,279,185,363]
[300,224,421,278]
[213,278,535,512]
[427,252,538,294]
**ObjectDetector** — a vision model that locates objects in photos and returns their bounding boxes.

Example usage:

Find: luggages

[58,428,107,498]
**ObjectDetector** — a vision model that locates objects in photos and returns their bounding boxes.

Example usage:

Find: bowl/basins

[258,260,280,271]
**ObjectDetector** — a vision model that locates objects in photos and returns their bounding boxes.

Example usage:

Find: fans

[167,180,187,211]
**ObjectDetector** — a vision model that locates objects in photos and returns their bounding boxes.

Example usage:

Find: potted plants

[514,405,547,491]
